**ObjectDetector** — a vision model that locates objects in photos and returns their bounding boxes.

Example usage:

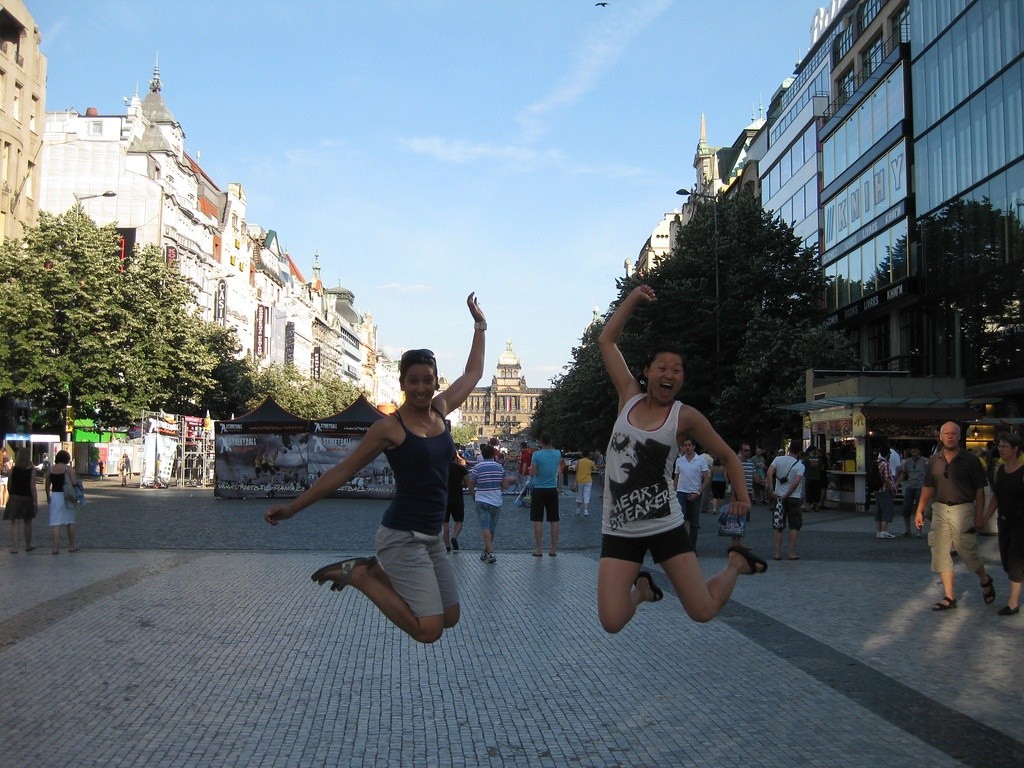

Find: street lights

[65,191,120,441]
[676,187,724,398]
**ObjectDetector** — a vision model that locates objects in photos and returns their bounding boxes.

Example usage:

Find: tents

[306,392,396,499]
[212,395,308,499]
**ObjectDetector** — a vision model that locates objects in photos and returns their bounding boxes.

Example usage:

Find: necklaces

[403,402,416,410]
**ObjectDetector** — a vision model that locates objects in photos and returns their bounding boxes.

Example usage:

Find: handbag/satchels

[717,504,746,536]
[570,481,578,492]
[62,469,78,510]
[772,497,785,530]
[725,483,732,495]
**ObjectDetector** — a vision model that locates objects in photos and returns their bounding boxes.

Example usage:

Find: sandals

[932,597,957,611]
[980,574,996,604]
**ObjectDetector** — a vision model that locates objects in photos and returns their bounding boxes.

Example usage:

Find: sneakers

[486,553,497,563]
[480,551,487,560]
[876,531,896,539]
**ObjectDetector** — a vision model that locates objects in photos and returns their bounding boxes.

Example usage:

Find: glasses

[401,348,435,362]
[997,445,1009,449]
[740,449,751,452]
[913,462,916,469]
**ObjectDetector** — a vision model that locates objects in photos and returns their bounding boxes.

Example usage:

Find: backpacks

[865,462,882,492]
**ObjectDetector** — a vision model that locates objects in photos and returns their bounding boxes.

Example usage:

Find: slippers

[531,551,543,557]
[548,551,557,556]
[775,556,783,560]
[789,554,800,560]
[727,546,768,576]
[634,571,663,602]
[51,550,59,554]
[68,547,80,552]
[311,555,378,592]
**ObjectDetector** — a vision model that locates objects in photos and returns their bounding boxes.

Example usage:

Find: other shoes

[998,605,1019,616]
[450,537,459,550]
[446,546,451,553]
[584,511,591,516]
[916,532,922,538]
[904,530,911,535]
[25,545,37,551]
[576,509,581,515]
[9,549,19,553]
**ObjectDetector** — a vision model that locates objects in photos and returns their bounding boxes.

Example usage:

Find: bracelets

[474,322,487,330]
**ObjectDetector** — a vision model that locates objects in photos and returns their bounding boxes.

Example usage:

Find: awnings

[776,397,981,411]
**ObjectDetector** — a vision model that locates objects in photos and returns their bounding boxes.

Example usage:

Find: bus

[565,452,596,460]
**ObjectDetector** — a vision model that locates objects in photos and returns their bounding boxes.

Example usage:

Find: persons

[265,291,488,644]
[674,437,827,559]
[913,421,996,609]
[118,453,132,486]
[597,286,769,634]
[45,449,81,553]
[981,417,1023,614]
[873,427,945,539]
[218,442,394,497]
[0,447,14,508]
[4,447,38,553]
[443,435,605,564]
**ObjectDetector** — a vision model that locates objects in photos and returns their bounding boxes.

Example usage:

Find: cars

[564,457,579,470]
